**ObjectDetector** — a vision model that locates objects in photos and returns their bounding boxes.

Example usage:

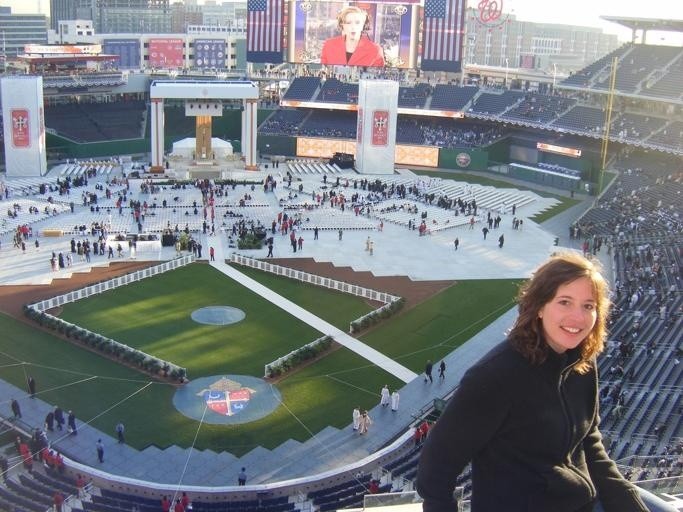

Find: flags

[244,1,284,65]
[418,0,464,72]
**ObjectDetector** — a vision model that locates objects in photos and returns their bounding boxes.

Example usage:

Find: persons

[160,494,170,511]
[390,389,399,413]
[26,374,35,398]
[179,492,190,511]
[438,360,446,379]
[422,359,433,382]
[52,404,64,431]
[10,398,21,418]
[418,420,429,440]
[414,254,683,511]
[115,420,124,444]
[319,6,386,67]
[0,62,578,271]
[237,466,246,485]
[351,405,360,431]
[565,44,681,418]
[45,412,54,430]
[379,384,390,409]
[368,478,380,495]
[173,498,184,512]
[94,438,104,461]
[67,409,77,433]
[415,428,422,443]
[358,410,371,434]
[0,425,87,512]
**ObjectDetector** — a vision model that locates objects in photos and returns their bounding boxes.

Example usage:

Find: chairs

[0,37,681,512]
